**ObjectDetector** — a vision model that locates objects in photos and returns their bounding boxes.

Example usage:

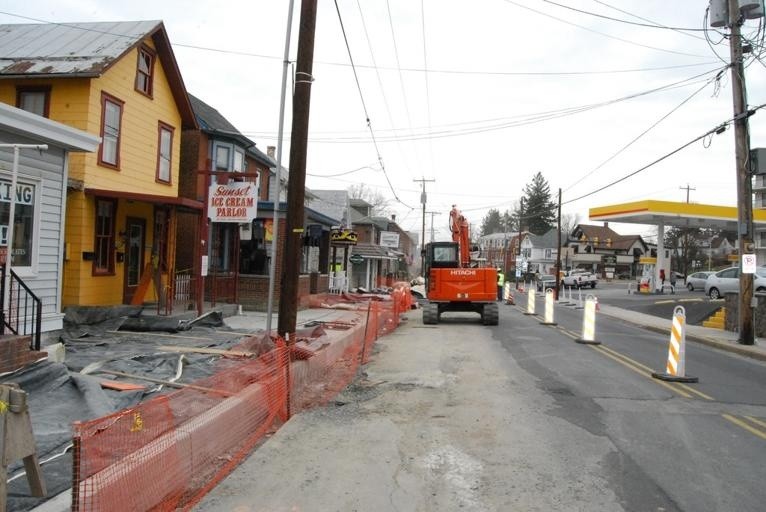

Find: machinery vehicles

[425,207,501,324]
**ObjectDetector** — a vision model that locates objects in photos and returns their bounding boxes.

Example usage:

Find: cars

[673,268,766,299]
[535,269,597,290]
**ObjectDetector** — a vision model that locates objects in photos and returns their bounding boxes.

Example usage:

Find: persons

[670,270,677,294]
[495,267,505,302]
